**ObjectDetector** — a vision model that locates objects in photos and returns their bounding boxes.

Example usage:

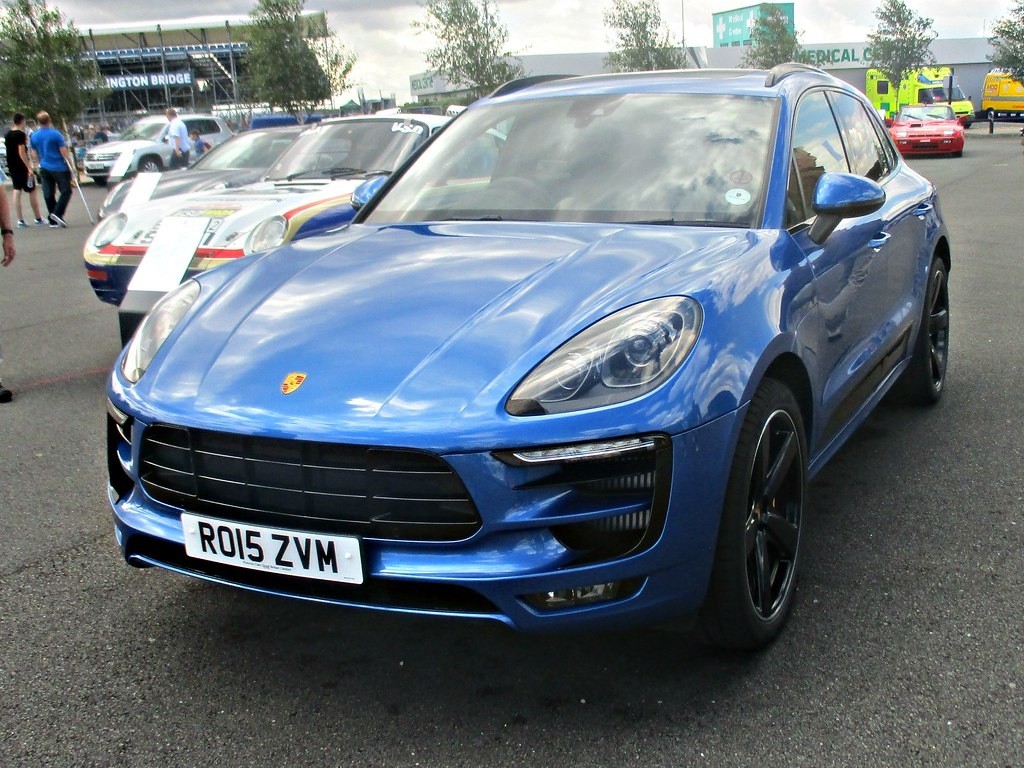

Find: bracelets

[1,228,14,235]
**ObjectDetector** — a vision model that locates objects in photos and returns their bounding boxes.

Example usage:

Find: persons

[165,109,191,170]
[30,111,77,227]
[6,114,49,228]
[189,129,208,156]
[24,119,133,145]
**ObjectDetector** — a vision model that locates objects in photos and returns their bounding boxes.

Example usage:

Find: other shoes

[0,384,13,403]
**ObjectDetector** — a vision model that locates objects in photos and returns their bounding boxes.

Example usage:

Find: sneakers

[34,217,50,226]
[16,219,30,228]
[48,211,68,227]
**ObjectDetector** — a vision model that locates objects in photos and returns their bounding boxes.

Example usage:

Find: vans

[866,68,974,129]
[979,68,1024,121]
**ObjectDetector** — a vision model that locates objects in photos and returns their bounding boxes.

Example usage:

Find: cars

[98,115,323,222]
[82,112,454,305]
[84,113,233,183]
[98,61,954,660]
[890,106,969,158]
